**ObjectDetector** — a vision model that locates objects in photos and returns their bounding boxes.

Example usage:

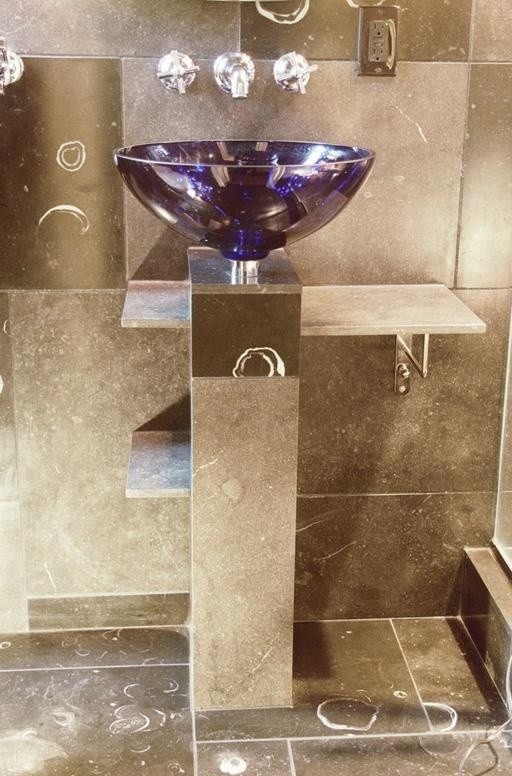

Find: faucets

[213,50,256,100]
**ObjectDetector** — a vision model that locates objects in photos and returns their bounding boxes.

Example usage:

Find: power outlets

[357,6,399,77]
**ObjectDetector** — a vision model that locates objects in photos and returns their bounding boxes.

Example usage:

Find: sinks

[114,139,377,262]
